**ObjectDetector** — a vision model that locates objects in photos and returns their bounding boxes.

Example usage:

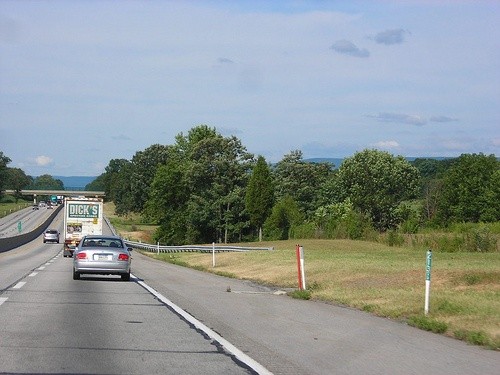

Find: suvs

[43,229,60,243]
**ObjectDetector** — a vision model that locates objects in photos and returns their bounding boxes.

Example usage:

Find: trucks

[51,195,63,206]
[64,197,103,257]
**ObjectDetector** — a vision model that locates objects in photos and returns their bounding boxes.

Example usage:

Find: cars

[33,204,39,210]
[72,234,133,280]
[39,201,53,209]
